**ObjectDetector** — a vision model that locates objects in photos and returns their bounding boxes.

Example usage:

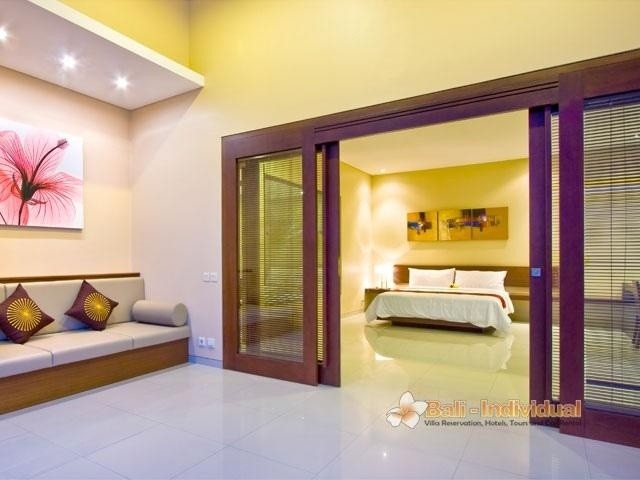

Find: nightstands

[365,288,390,312]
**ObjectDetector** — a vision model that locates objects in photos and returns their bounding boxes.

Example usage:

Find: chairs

[630,280,640,349]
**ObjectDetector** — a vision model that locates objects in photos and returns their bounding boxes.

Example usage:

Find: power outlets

[203,271,210,282]
[197,336,207,348]
[206,337,216,348]
[210,272,218,282]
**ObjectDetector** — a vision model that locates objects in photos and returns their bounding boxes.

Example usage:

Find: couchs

[0,273,191,415]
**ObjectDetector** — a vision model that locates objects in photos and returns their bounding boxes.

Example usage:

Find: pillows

[132,300,189,327]
[408,267,455,289]
[63,280,119,331]
[0,284,55,345]
[453,269,508,292]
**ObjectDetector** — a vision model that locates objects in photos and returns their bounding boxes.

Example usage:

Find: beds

[363,265,560,337]
